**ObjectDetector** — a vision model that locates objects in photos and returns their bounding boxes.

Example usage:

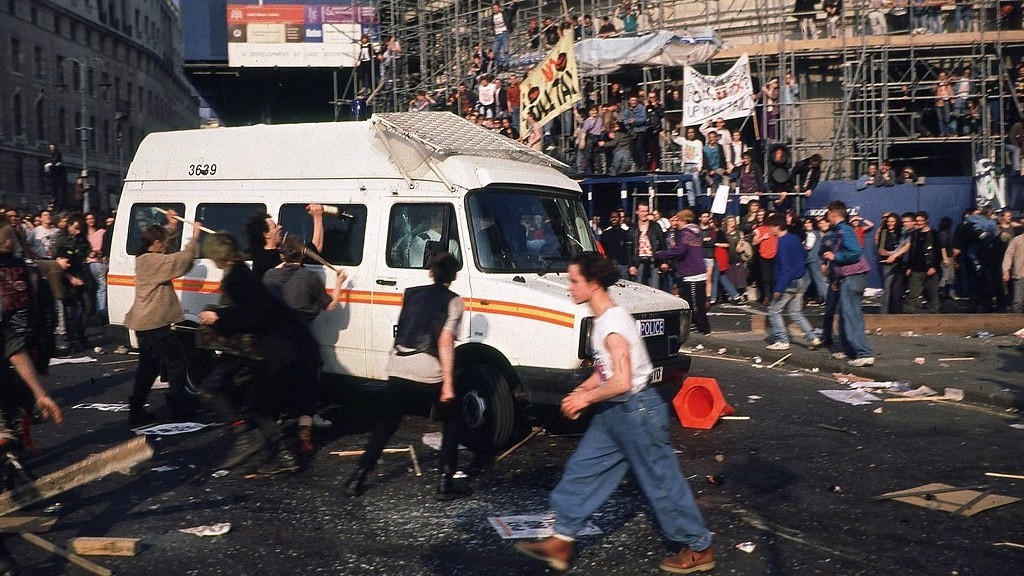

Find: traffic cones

[672,377,737,430]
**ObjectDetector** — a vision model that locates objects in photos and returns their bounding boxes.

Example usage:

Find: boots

[344,461,368,496]
[128,396,156,427]
[437,473,473,499]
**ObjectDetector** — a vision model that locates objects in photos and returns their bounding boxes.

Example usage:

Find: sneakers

[512,535,574,571]
[658,547,716,574]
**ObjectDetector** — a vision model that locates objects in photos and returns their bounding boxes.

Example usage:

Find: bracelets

[573,386,586,393]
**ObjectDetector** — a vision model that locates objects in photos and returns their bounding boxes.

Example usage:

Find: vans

[106,120,694,456]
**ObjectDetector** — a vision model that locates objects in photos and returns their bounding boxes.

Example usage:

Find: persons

[515,251,714,573]
[123,210,200,425]
[0,201,170,511]
[75,177,91,211]
[196,202,347,472]
[45,142,64,210]
[408,0,1024,368]
[345,252,473,498]
[356,35,403,94]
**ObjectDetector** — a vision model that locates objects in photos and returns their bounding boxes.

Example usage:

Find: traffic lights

[766,140,791,190]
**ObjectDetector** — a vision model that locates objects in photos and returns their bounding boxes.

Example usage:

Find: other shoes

[296,441,314,456]
[71,338,91,351]
[221,427,267,470]
[257,450,301,475]
[808,338,822,347]
[766,342,789,351]
[832,352,849,359]
[847,357,875,366]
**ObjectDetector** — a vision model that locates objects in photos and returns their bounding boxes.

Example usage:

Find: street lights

[52,54,114,215]
